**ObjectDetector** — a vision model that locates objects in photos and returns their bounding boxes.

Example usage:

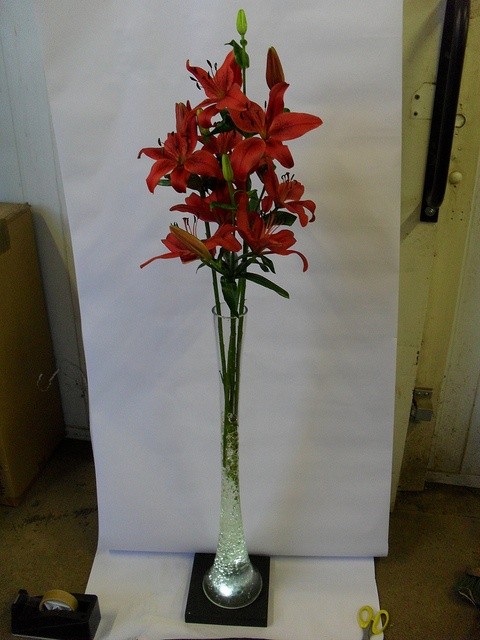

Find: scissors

[358,605,389,640]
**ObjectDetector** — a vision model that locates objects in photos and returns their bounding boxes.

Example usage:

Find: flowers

[137,9,324,472]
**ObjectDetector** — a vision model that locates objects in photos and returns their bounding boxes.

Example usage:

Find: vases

[203,303,262,609]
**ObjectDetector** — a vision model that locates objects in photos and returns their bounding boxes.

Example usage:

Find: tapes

[39,590,78,612]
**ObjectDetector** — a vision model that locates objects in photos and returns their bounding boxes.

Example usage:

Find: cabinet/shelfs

[0,203,64,505]
